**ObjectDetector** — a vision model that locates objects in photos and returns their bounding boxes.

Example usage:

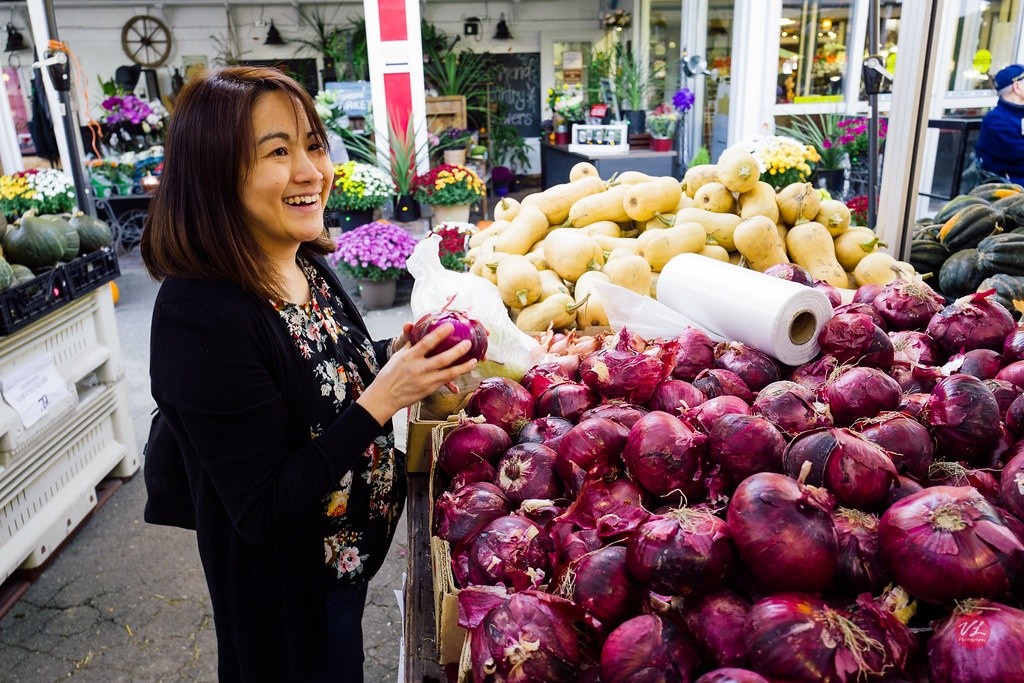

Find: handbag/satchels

[143,408,199,531]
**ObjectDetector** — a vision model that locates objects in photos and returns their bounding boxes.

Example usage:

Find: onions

[411,262,1024,683]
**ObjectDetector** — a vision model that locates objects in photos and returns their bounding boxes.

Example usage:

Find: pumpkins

[0,205,111,291]
[911,183,1024,329]
[109,280,119,305]
[468,146,917,335]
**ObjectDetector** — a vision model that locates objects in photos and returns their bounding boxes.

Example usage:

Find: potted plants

[774,108,852,192]
[334,106,452,224]
[595,42,682,139]
[284,1,353,88]
[481,102,533,195]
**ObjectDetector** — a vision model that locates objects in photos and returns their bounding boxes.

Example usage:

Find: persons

[141,66,480,681]
[977,64,1024,183]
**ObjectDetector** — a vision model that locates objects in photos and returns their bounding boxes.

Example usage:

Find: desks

[539,142,677,192]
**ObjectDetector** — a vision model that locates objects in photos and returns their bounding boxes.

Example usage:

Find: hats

[993,64,1024,91]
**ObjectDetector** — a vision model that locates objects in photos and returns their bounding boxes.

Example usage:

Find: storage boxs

[66,247,122,298]
[0,268,71,339]
[402,396,486,664]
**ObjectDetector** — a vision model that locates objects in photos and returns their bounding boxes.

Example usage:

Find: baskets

[1,243,121,340]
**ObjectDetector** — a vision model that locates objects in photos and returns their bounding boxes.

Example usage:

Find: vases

[432,204,469,229]
[116,182,133,196]
[358,278,396,306]
[653,138,670,151]
[342,210,373,230]
[92,182,111,196]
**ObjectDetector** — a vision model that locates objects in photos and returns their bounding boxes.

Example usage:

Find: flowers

[0,0,896,311]
